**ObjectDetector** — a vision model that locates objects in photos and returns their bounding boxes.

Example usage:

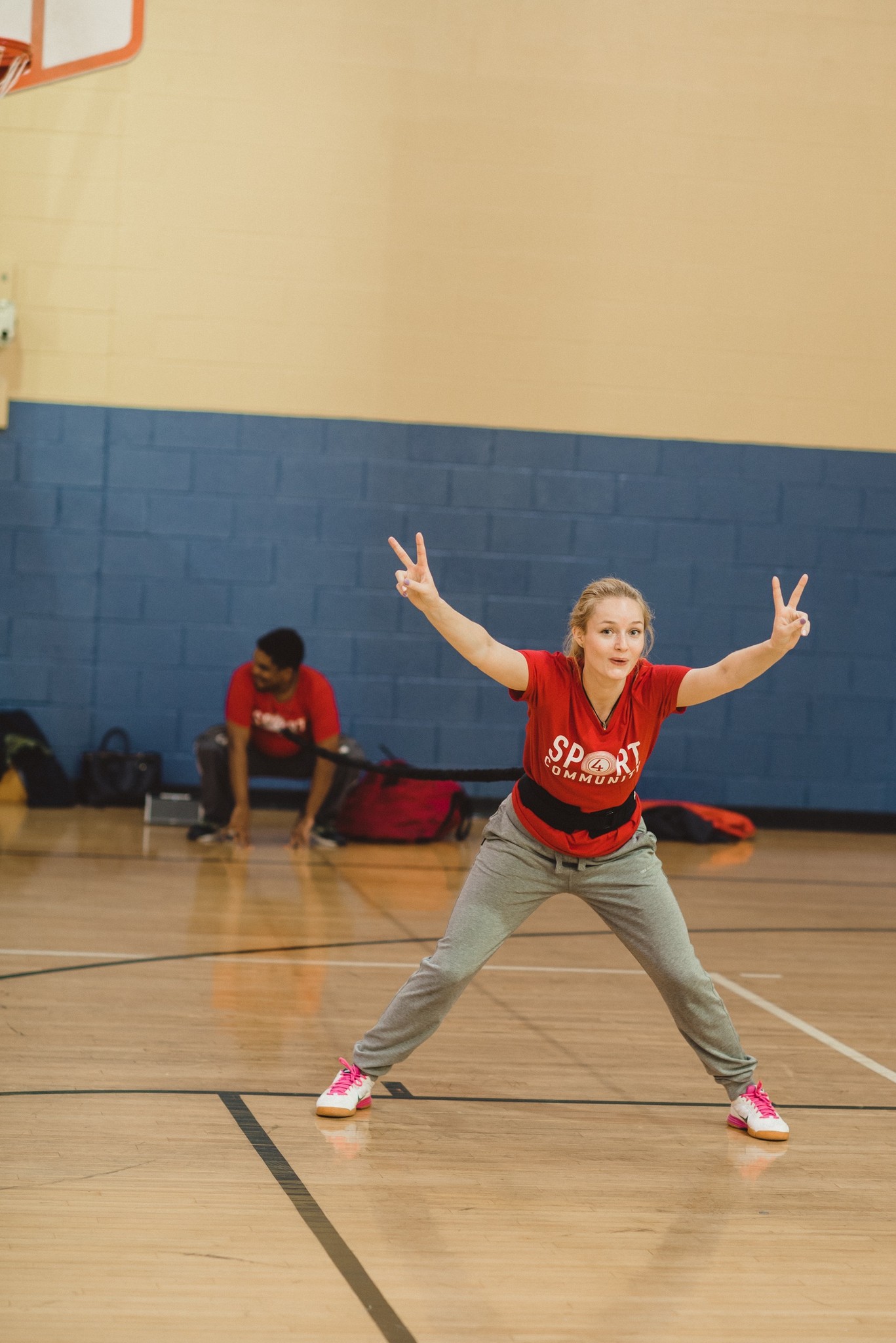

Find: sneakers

[315,1056,376,1117]
[727,1126,787,1179]
[727,1079,789,1140]
[316,1110,370,1152]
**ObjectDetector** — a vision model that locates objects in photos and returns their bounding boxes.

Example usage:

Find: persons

[184,626,352,856]
[315,529,812,1141]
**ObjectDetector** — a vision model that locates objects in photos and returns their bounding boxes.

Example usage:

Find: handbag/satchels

[78,724,162,807]
[322,756,474,842]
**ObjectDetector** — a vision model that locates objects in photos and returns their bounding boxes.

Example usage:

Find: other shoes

[187,820,230,843]
[291,814,346,848]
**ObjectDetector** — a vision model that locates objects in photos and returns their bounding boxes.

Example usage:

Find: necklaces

[581,665,625,731]
